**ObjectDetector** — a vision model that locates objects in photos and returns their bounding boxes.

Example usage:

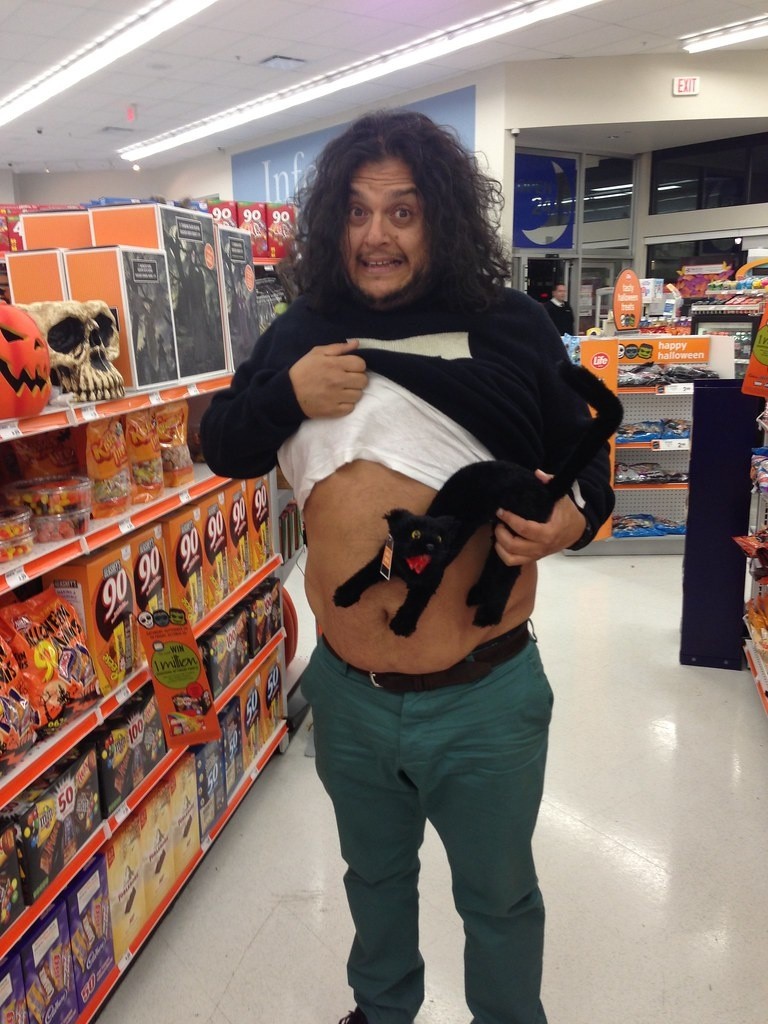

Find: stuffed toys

[329,366,623,638]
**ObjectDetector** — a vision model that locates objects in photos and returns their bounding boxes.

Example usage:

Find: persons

[543,282,576,336]
[193,111,621,1023]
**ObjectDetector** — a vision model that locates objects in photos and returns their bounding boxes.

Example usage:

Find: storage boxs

[0,196,296,1021]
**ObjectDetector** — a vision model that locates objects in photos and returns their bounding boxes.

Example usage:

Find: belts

[321,620,529,693]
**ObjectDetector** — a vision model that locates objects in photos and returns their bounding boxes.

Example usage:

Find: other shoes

[338,1007,368,1024]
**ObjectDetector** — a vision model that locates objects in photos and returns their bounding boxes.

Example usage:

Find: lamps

[677,15,768,58]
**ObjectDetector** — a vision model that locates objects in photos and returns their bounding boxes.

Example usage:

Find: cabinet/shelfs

[613,376,690,541]
[1,367,299,1024]
[742,413,768,711]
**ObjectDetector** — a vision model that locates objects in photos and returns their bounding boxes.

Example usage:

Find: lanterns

[0,294,52,421]
